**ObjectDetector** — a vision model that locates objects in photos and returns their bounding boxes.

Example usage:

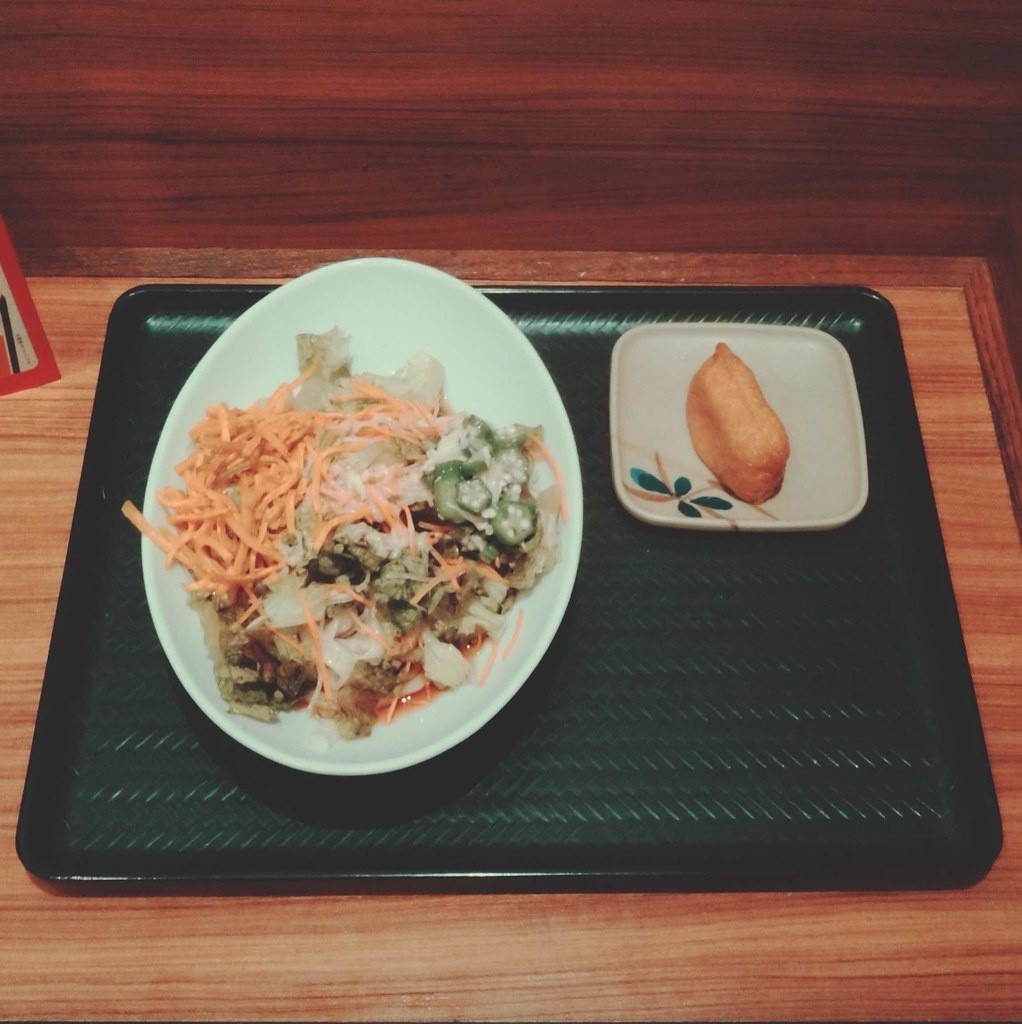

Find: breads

[686,343,789,503]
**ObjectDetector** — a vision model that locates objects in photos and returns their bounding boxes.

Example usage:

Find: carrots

[121,364,570,721]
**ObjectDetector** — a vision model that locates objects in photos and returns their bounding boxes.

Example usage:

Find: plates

[610,321,869,532]
[140,259,587,779]
